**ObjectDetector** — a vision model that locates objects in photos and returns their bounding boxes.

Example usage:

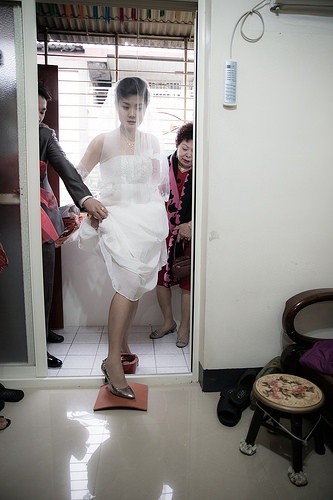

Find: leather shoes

[47,351,62,368]
[47,329,64,343]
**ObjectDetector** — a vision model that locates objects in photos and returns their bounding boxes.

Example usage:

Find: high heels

[101,356,135,399]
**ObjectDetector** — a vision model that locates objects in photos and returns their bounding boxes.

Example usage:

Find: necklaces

[121,131,142,148]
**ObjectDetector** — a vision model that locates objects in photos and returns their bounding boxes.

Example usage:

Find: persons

[75,75,171,401]
[149,121,193,348]
[0,244,25,412]
[38,85,108,369]
[0,415,11,431]
[47,204,70,344]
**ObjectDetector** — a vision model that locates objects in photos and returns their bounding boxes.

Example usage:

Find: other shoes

[176,329,190,348]
[150,323,177,339]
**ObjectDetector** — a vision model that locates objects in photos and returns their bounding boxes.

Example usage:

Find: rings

[100,206,104,210]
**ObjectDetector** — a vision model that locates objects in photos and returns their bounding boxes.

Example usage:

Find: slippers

[217,370,258,427]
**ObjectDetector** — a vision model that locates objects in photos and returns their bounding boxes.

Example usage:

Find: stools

[239,373,326,487]
